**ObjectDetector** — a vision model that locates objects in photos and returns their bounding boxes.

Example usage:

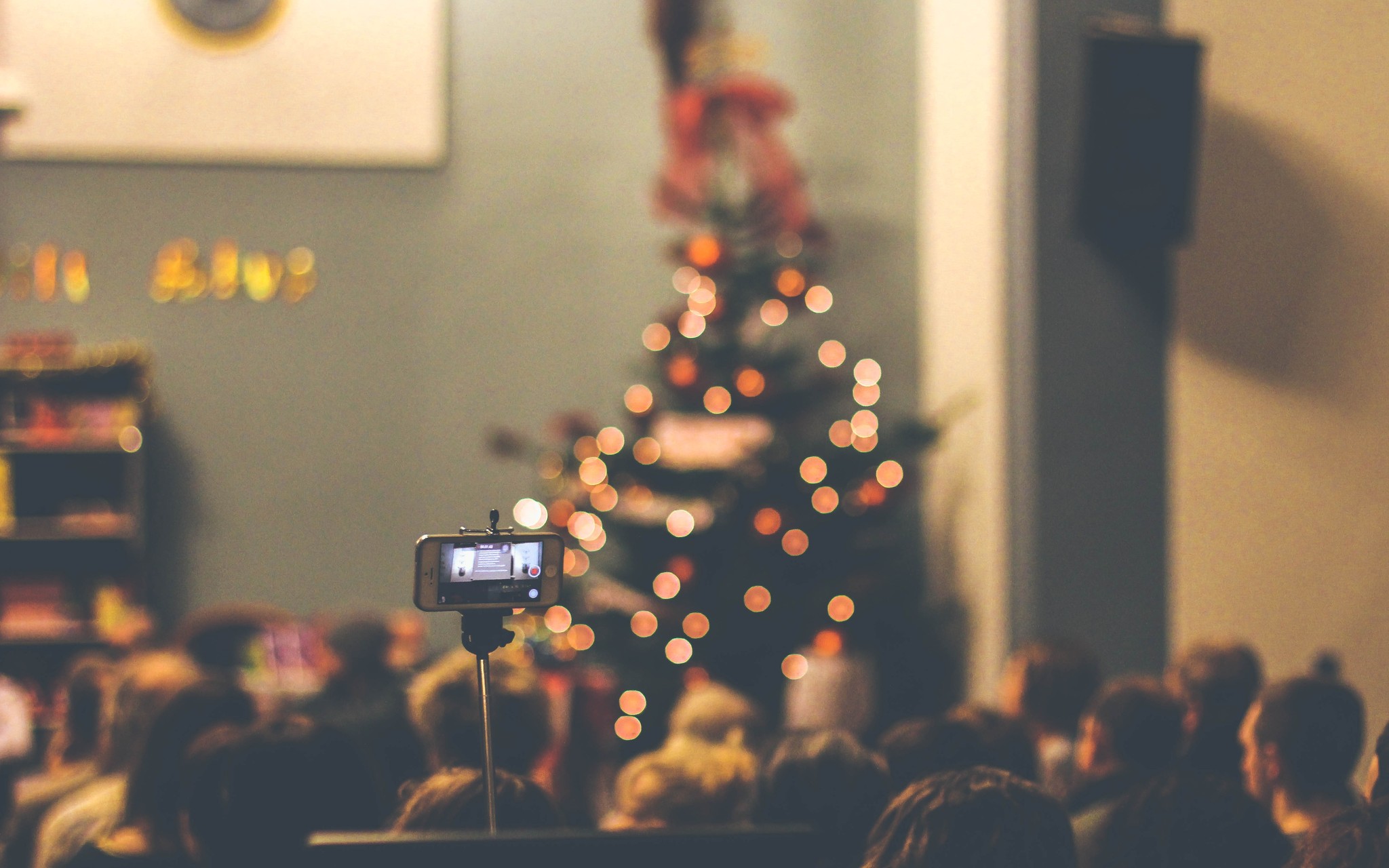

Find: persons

[0,601,1389,867]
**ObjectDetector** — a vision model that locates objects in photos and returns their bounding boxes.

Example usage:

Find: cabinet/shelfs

[0,341,159,654]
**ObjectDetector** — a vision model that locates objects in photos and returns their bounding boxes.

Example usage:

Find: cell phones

[413,532,565,611]
